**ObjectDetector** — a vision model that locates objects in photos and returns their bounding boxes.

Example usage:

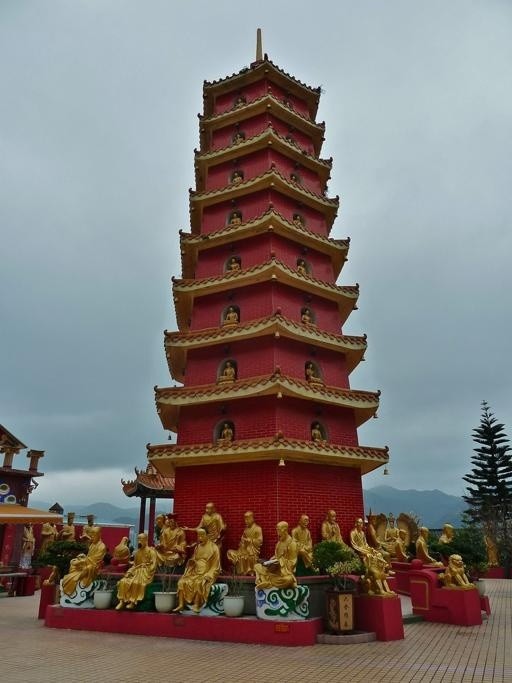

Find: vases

[93,588,114,609]
[152,590,177,613]
[222,594,247,618]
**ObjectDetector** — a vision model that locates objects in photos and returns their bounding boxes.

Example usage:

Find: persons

[21,502,187,610]
[172,528,221,613]
[321,509,344,542]
[227,510,264,576]
[220,96,327,442]
[350,508,502,595]
[291,514,320,573]
[254,520,297,588]
[183,500,228,549]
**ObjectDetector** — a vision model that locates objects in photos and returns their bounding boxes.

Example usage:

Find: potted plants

[42,539,90,605]
[312,536,369,638]
